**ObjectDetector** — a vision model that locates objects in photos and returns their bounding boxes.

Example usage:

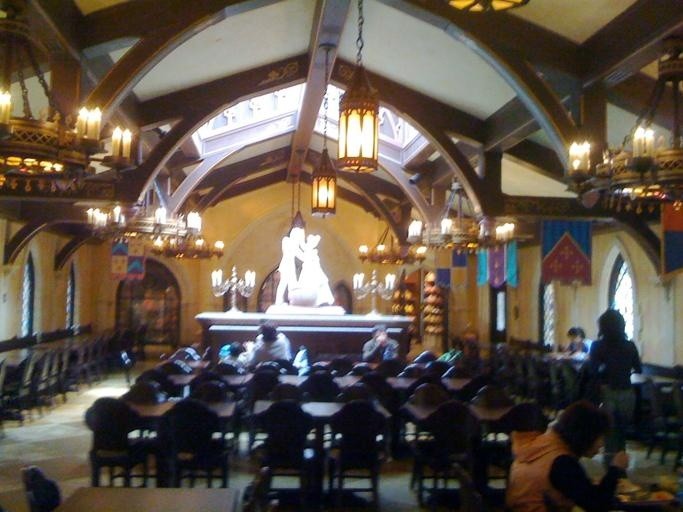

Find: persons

[361,323,401,365]
[583,306,643,477]
[272,224,336,307]
[217,319,291,371]
[434,336,465,363]
[503,398,631,512]
[561,323,589,355]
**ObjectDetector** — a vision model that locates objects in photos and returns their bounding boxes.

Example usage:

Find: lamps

[0,0,135,197]
[337,0,382,176]
[357,180,515,264]
[311,43,337,218]
[562,47,683,212]
[288,149,305,242]
[211,265,256,314]
[86,174,224,258]
[353,270,396,316]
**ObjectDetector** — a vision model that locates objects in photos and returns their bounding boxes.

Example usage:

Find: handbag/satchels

[292,344,308,368]
[436,348,464,364]
